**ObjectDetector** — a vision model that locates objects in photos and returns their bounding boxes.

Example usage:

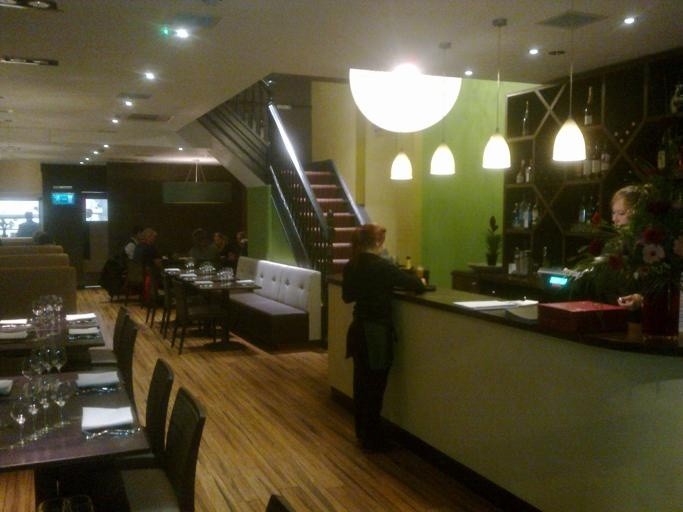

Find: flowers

[553,157,682,310]
[482,215,503,255]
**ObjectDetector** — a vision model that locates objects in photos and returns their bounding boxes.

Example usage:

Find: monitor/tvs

[81,193,110,226]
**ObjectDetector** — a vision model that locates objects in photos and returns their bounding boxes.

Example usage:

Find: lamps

[386,0,588,182]
[160,159,232,206]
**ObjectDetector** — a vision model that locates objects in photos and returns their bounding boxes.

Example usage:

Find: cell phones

[547,275,567,286]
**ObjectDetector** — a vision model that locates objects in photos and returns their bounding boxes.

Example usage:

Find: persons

[15,212,41,235]
[120,224,250,311]
[343,223,425,452]
[32,230,52,243]
[549,182,666,327]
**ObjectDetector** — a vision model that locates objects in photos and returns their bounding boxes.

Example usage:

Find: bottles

[522,97,532,137]
[502,122,668,279]
[583,85,599,126]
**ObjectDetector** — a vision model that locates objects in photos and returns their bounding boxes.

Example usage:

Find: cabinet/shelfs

[504,45,683,269]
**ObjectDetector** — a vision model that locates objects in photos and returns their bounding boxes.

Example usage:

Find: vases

[640,288,678,334]
[485,254,498,265]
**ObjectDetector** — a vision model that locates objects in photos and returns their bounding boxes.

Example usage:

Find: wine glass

[8,294,68,448]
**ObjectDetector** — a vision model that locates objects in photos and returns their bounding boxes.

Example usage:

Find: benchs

[228,256,321,344]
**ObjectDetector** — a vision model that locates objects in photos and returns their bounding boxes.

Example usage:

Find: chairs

[109,250,219,355]
